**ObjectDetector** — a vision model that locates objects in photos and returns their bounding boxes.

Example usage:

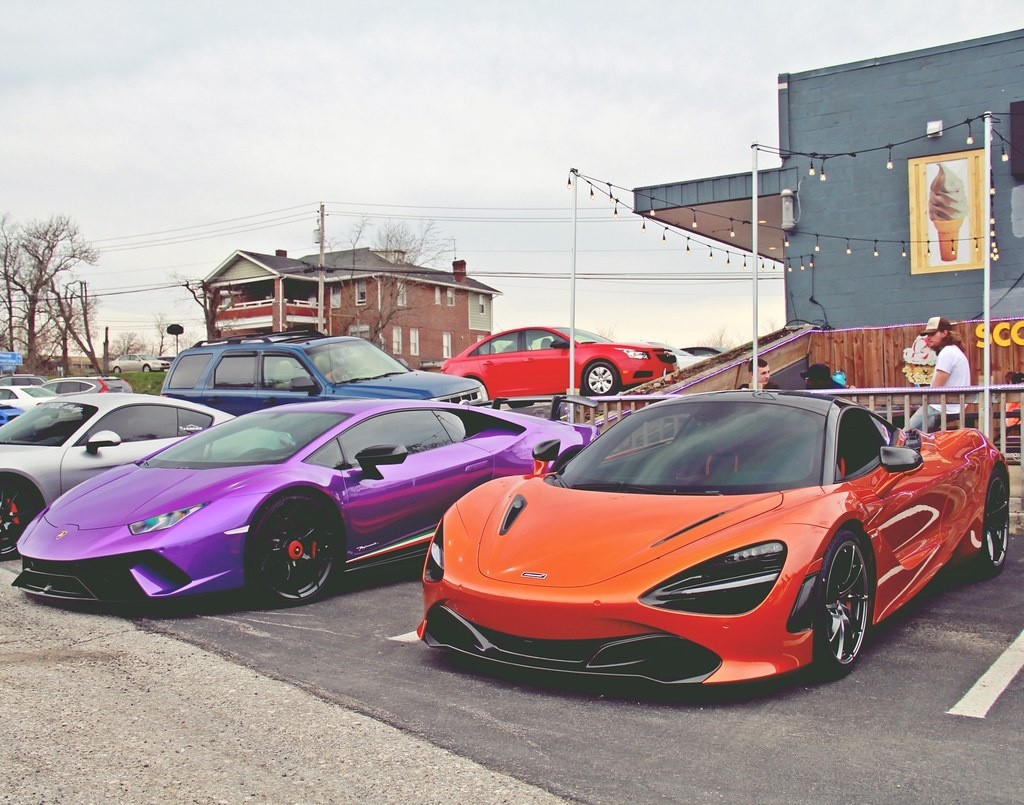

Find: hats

[832,370,845,385]
[920,317,950,336]
[800,363,829,380]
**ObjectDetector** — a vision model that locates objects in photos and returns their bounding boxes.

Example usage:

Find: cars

[0,385,62,410]
[107,354,170,373]
[0,403,24,428]
[440,325,677,408]
[638,340,728,373]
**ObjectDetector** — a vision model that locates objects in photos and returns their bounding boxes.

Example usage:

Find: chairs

[274,360,294,388]
[540,338,553,349]
[313,356,332,374]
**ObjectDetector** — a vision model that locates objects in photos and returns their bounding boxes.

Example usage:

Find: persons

[901,317,970,433]
[1004,371,1024,426]
[737,358,779,390]
[799,364,846,390]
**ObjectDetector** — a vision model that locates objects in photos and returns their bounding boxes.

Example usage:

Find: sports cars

[416,385,1011,684]
[0,393,298,561]
[12,395,601,610]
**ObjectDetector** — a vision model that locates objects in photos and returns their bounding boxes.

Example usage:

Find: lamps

[781,189,796,233]
[926,120,943,137]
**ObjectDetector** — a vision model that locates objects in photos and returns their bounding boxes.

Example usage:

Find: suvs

[159,331,489,418]
[41,377,133,393]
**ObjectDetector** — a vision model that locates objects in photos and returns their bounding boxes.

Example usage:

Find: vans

[0,373,46,386]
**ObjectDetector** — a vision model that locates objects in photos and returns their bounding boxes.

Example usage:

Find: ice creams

[926,163,967,261]
[900,334,939,385]
